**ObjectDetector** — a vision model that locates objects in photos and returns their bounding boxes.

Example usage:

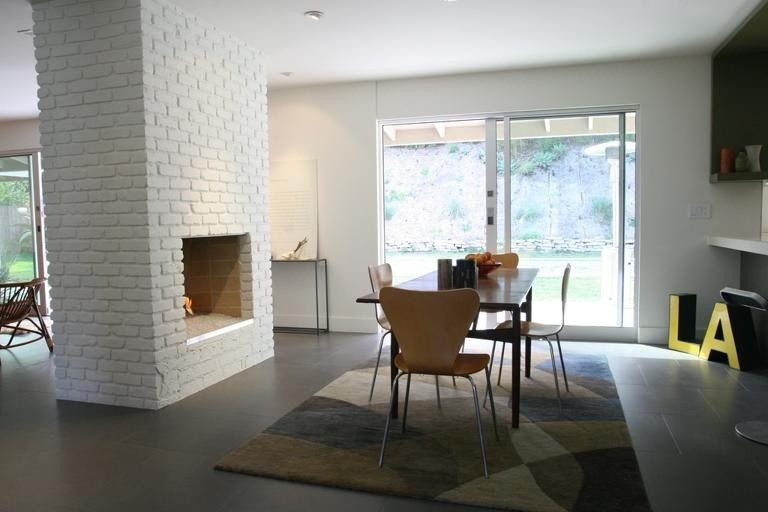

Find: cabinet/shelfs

[709,1,768,184]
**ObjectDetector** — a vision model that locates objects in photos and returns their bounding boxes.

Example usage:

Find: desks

[356,267,540,429]
[273,259,328,336]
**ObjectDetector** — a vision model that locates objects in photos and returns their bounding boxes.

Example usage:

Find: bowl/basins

[477,262,502,275]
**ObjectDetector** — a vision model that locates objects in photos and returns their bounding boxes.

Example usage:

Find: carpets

[215,340,653,512]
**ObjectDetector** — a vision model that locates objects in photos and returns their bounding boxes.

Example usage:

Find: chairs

[377,286,499,480]
[0,278,53,366]
[368,249,519,401]
[482,264,572,410]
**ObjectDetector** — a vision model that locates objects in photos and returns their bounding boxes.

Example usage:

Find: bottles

[735,152,751,172]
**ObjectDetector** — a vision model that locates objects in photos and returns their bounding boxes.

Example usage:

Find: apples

[466,252,496,265]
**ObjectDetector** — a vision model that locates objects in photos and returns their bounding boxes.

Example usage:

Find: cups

[437,258,453,290]
